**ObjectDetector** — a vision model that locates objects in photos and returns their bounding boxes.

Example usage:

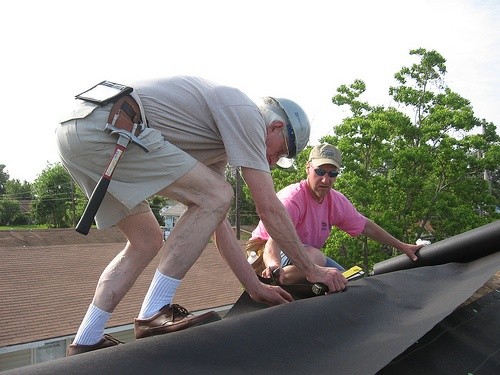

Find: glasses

[310,165,340,177]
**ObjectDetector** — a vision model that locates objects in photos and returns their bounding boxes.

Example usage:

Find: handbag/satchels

[245,237,266,278]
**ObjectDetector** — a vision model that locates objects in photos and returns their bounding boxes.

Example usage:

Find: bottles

[247,251,258,264]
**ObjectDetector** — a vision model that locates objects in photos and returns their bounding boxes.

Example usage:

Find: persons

[54,74,349,356]
[246,144,425,286]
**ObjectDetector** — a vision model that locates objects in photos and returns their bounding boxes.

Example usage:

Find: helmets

[265,97,310,158]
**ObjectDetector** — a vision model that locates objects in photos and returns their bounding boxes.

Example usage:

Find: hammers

[75,122,150,236]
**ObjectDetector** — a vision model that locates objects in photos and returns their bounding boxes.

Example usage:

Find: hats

[308,144,341,169]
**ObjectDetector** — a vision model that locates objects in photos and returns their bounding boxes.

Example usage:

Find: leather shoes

[134,305,222,339]
[66,333,125,358]
